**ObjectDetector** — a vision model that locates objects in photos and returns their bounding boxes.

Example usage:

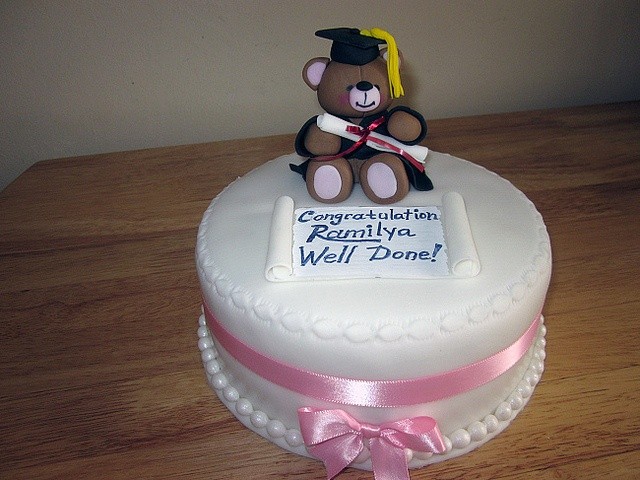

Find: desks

[0,100,640,479]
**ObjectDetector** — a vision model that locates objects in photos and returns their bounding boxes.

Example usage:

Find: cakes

[195,29,552,468]
